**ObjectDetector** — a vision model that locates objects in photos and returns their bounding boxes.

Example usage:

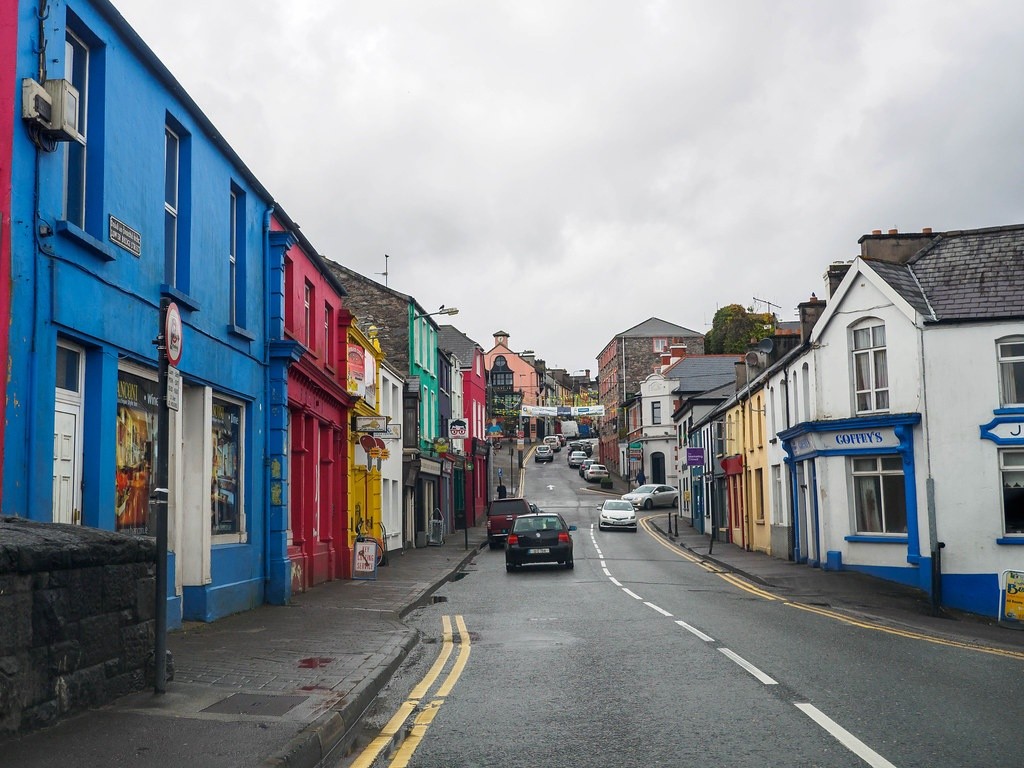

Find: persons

[496,483,506,499]
[635,469,646,486]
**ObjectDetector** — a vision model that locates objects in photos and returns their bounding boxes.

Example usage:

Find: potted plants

[600,477,615,489]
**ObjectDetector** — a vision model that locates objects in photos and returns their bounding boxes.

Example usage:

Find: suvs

[535,445,554,462]
[543,435,562,451]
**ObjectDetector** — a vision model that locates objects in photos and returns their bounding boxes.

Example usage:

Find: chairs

[519,522,532,529]
[545,521,558,530]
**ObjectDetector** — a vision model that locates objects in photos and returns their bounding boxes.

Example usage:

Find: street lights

[572,370,584,407]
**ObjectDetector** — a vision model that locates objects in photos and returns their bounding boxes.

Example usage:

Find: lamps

[414,307,460,320]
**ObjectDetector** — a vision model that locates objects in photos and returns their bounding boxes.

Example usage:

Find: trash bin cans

[427,507,445,547]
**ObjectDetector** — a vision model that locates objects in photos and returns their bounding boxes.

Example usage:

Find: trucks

[570,441,592,455]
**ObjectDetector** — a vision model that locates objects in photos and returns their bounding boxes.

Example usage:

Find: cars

[568,450,588,469]
[502,513,577,571]
[528,503,544,523]
[597,499,639,531]
[556,434,565,446]
[583,464,609,481]
[487,498,533,549]
[579,459,596,475]
[621,484,679,509]
